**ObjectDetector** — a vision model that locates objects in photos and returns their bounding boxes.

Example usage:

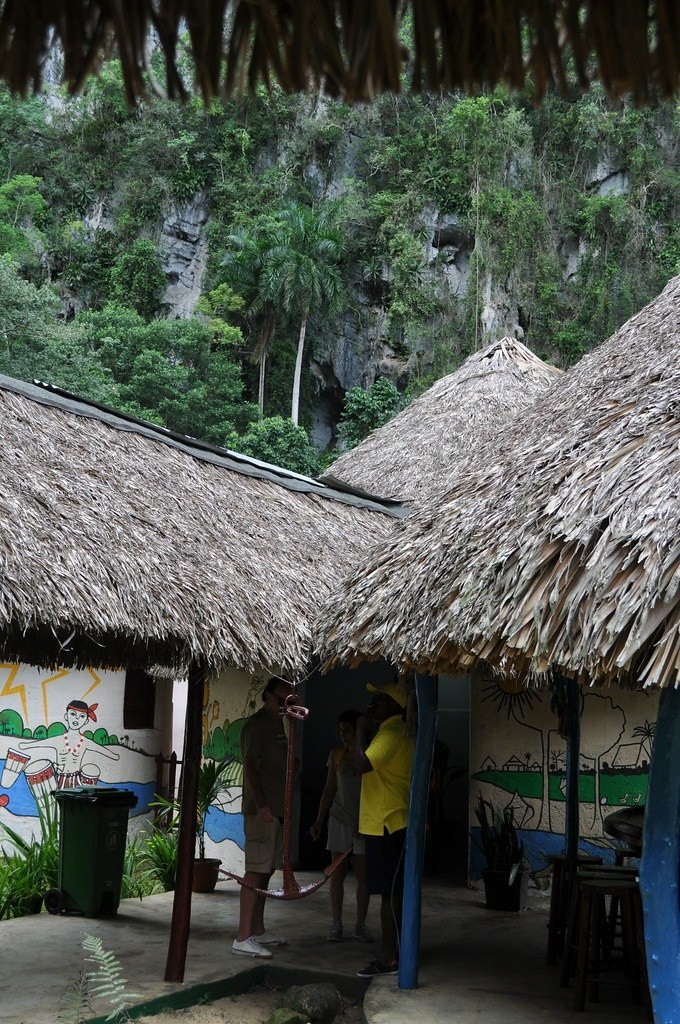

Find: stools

[542,853,647,1015]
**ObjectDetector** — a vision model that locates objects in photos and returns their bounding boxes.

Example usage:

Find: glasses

[270,691,295,706]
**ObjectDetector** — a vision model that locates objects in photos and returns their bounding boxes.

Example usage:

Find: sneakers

[357,951,399,978]
[253,930,287,946]
[353,924,380,942]
[231,937,273,959]
[326,922,343,942]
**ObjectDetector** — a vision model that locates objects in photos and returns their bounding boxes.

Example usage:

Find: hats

[366,678,409,709]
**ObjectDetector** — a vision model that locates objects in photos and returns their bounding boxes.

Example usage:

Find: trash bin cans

[42,786,140,918]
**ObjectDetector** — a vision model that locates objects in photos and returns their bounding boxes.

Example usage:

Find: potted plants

[0,832,55,918]
[466,788,531,915]
[146,752,240,894]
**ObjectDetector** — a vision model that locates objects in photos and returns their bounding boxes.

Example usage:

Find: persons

[230,674,303,959]
[331,679,417,977]
[311,705,380,944]
